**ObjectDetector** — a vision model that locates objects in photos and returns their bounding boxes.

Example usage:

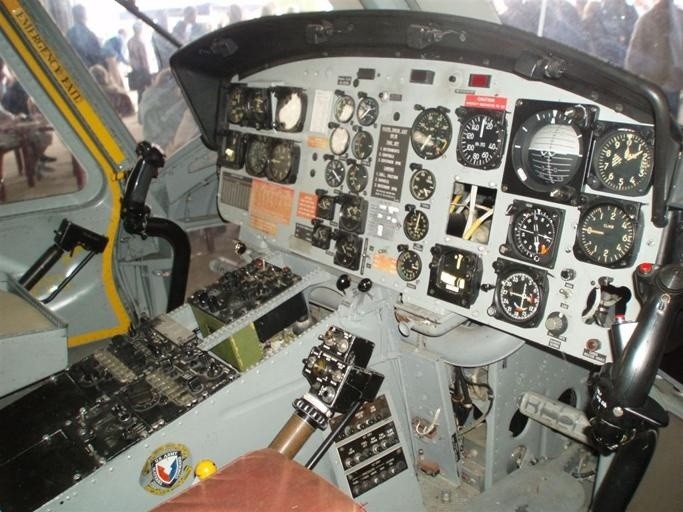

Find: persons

[0,3,302,173]
[495,0,682,121]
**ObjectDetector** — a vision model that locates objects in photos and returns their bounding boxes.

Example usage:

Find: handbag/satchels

[128,72,138,92]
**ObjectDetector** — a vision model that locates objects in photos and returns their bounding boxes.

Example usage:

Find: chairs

[0,127,37,202]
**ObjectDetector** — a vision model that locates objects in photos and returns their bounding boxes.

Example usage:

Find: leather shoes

[38,155,58,172]
